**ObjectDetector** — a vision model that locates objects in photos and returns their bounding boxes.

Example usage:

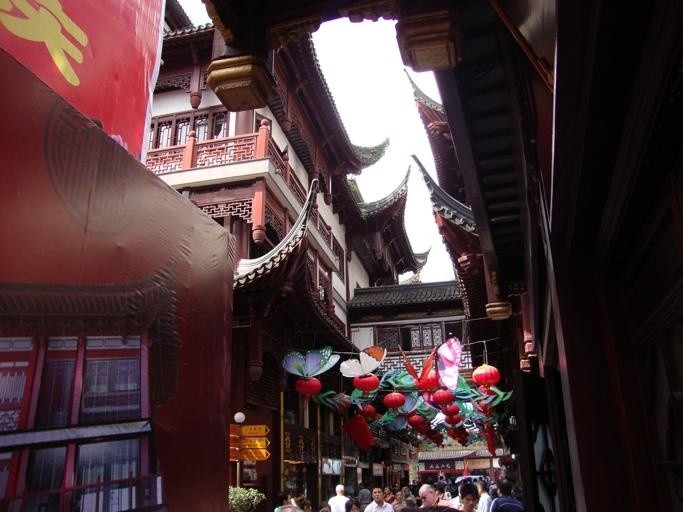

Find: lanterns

[293,363,501,458]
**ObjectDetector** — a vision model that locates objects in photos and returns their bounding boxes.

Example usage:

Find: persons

[271,470,523,511]
